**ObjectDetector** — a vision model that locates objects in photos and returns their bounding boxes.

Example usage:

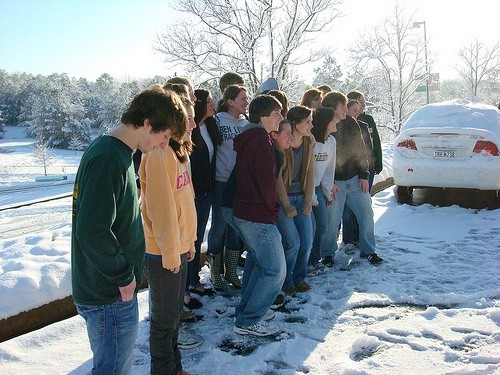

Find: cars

[392,99,500,208]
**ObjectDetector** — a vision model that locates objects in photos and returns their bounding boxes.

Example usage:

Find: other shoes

[274,295,284,305]
[297,282,311,292]
[184,297,201,309]
[182,309,195,321]
[189,288,215,297]
[307,265,319,276]
[344,244,356,253]
[283,286,297,296]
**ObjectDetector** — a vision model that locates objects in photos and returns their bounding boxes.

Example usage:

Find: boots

[210,251,227,291]
[224,247,242,289]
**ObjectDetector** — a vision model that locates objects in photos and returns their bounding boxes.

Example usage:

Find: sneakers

[361,252,384,264]
[263,307,275,320]
[177,328,204,348]
[323,256,334,266]
[232,319,279,336]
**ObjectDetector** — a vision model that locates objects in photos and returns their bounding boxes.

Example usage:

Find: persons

[218,72,244,111]
[208,85,249,291]
[221,94,287,336]
[71,88,187,375]
[342,91,383,254]
[132,77,203,348]
[271,119,292,148]
[307,107,336,275]
[185,89,220,309]
[321,93,383,268]
[276,105,317,292]
[259,78,289,117]
[302,86,331,109]
[139,96,197,375]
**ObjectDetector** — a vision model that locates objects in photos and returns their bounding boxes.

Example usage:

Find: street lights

[413,20,430,106]
